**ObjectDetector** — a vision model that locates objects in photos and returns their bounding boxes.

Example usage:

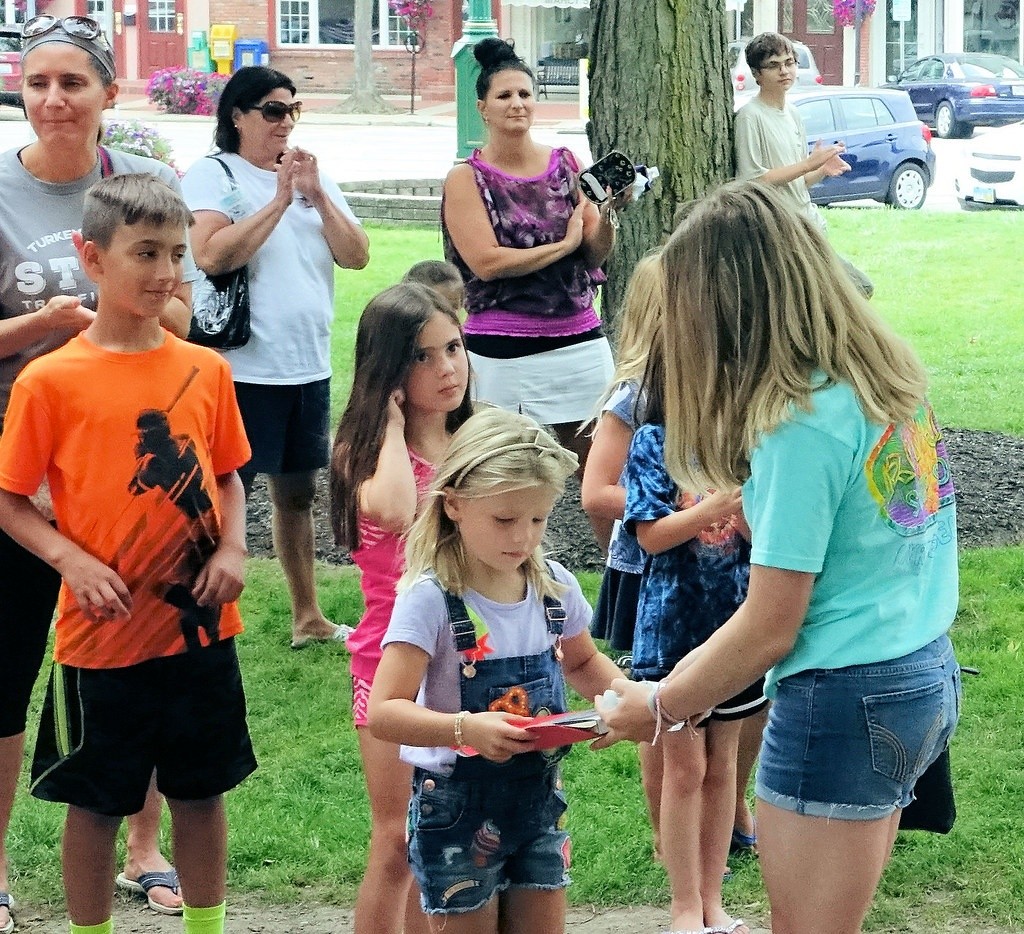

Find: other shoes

[731,820,758,853]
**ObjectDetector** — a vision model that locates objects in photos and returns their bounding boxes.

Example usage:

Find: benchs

[535,59,580,100]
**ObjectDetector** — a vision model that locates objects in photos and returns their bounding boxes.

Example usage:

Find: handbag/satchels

[185,158,251,349]
[897,748,956,834]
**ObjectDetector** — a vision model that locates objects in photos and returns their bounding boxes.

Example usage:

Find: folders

[451,708,609,758]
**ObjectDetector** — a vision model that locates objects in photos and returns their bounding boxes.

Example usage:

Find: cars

[0,24,28,120]
[878,52,1024,138]
[722,39,823,92]
[956,118,1024,212]
[736,85,936,212]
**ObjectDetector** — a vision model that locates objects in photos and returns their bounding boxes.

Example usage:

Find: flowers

[833,0,876,27]
[388,0,433,23]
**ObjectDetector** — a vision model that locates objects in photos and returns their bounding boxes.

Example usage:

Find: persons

[0,17,185,934]
[734,33,852,241]
[0,171,258,934]
[330,246,772,934]
[651,175,962,934]
[435,37,620,558]
[178,67,357,650]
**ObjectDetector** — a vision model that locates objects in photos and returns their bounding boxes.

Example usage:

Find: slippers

[0,893,15,934]
[291,623,356,649]
[117,869,185,915]
[703,914,752,934]
[667,918,707,934]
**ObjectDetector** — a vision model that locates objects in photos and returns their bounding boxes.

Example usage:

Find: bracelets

[646,684,688,728]
[454,710,471,747]
[654,684,698,746]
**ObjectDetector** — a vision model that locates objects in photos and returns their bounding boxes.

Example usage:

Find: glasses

[249,101,302,124]
[20,15,115,59]
[757,59,800,72]
